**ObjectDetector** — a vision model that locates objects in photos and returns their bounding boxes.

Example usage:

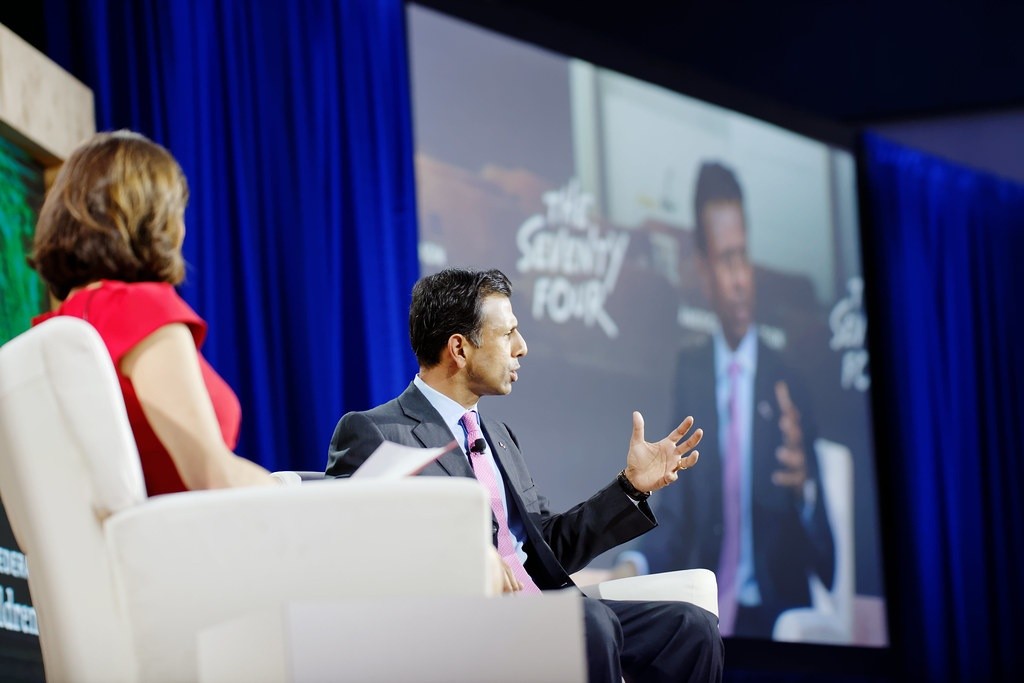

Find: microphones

[471,438,486,454]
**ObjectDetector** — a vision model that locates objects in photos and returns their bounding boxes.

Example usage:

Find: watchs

[617,469,652,502]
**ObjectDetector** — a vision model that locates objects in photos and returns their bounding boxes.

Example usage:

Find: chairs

[772,438,856,644]
[0,315,720,683]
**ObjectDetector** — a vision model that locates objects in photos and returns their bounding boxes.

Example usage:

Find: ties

[716,362,743,636]
[462,411,541,592]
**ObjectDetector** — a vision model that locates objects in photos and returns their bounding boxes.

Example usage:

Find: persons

[602,162,835,641]
[27,133,274,498]
[323,269,725,683]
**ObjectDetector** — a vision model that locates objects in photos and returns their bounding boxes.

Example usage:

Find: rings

[677,458,687,471]
